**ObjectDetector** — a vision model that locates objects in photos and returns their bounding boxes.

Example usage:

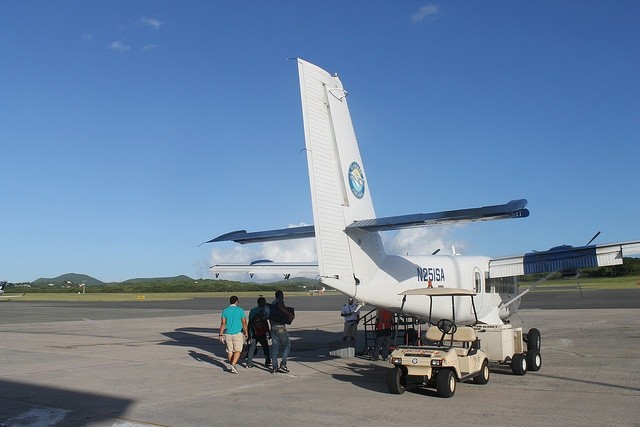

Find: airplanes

[197,57,637,327]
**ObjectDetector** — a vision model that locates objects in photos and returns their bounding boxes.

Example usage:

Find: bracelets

[219,334,223,336]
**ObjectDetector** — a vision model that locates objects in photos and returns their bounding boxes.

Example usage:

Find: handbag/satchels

[263,302,295,324]
[251,312,269,338]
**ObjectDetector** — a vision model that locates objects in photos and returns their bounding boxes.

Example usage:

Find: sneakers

[230,365,238,374]
[279,367,289,372]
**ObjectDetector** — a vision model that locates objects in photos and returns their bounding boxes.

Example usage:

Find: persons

[219,296,247,374]
[341,297,360,347]
[245,297,273,369]
[372,308,395,362]
[269,290,293,374]
